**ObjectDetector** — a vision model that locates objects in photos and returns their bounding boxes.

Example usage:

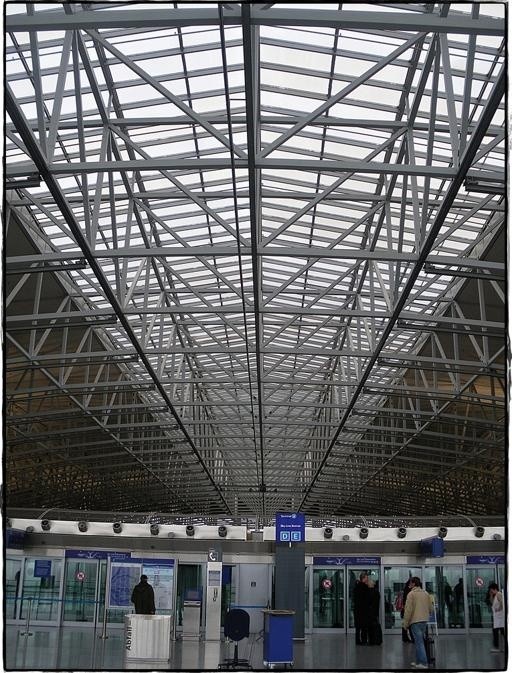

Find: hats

[140,575,148,579]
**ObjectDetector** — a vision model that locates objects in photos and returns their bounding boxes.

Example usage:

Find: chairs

[217,609,254,669]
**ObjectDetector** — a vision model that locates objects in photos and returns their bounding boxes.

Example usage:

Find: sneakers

[410,661,428,669]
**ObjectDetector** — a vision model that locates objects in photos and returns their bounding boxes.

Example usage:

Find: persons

[442,575,471,627]
[399,577,414,643]
[385,589,404,628]
[401,576,433,668]
[351,571,383,647]
[131,574,155,615]
[318,571,357,627]
[487,582,504,653]
